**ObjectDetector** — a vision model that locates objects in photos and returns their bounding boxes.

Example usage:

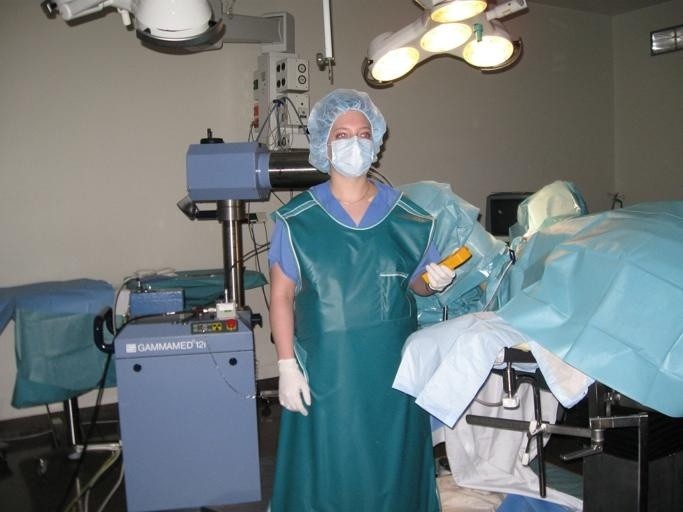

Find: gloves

[424,262,456,291]
[278,358,311,416]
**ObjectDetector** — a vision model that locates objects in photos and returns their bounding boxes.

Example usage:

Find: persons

[264,87,455,511]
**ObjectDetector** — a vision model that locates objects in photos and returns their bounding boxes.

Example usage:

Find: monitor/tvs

[486,193,534,241]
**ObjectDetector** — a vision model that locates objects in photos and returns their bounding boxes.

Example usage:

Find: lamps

[359,0,529,89]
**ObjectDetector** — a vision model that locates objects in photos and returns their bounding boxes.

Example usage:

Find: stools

[13,279,118,484]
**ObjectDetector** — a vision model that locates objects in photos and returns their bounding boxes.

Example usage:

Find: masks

[328,136,375,176]
